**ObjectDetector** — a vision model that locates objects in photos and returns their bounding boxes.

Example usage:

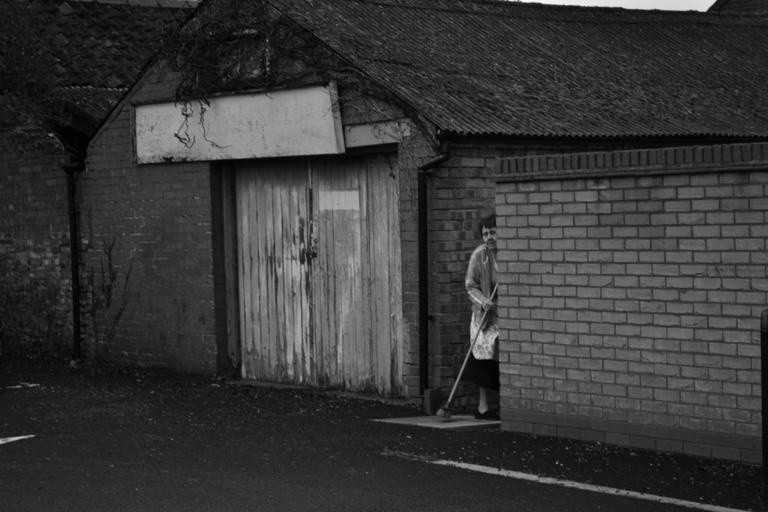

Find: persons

[465,214,498,419]
